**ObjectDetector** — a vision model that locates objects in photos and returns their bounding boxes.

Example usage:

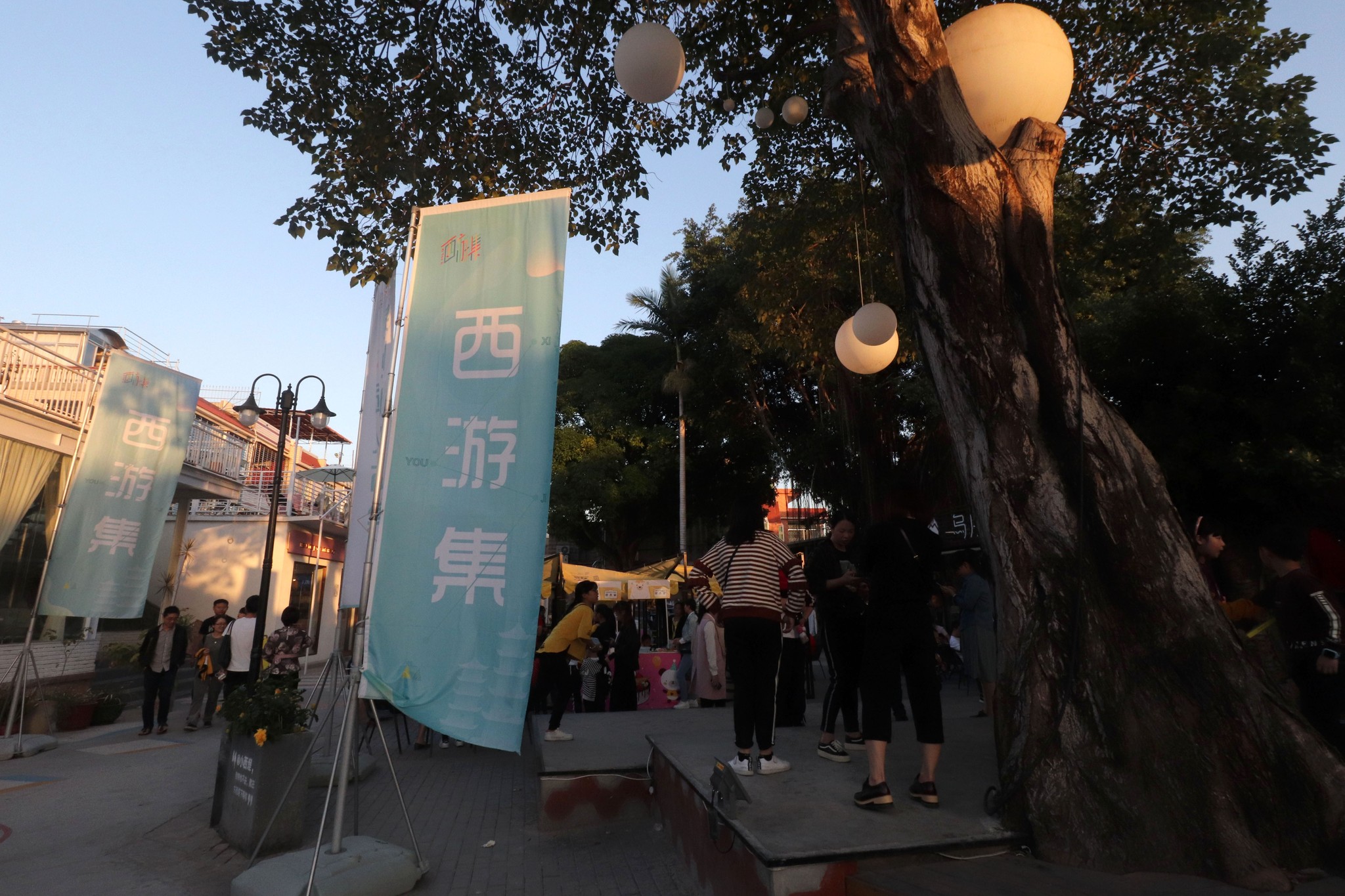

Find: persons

[135,606,187,737]
[184,594,311,732]
[1185,511,1345,763]
[688,478,993,813]
[302,494,338,515]
[525,580,728,740]
[413,726,465,748]
[267,476,298,516]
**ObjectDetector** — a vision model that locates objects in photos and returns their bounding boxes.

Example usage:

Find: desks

[604,651,681,709]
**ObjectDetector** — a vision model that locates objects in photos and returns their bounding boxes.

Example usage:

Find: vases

[209,724,315,858]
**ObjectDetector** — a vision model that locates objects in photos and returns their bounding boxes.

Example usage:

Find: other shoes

[414,742,426,750]
[854,774,895,814]
[728,755,754,776]
[908,773,938,809]
[673,700,690,710]
[138,728,153,736]
[183,724,198,731]
[979,695,984,704]
[156,724,168,734]
[204,721,212,728]
[687,699,699,707]
[895,715,909,721]
[756,755,791,775]
[456,741,463,746]
[439,741,449,748]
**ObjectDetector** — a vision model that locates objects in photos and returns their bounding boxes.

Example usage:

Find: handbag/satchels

[218,620,235,667]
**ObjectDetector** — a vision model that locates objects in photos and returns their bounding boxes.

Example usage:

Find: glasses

[215,623,227,626]
[590,589,598,592]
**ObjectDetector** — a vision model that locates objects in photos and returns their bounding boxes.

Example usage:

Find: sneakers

[817,739,851,762]
[844,735,866,750]
[544,728,573,741]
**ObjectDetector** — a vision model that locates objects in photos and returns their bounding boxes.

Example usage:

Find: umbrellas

[295,464,354,504]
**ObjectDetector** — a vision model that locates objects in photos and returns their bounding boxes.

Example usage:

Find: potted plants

[19,627,126,735]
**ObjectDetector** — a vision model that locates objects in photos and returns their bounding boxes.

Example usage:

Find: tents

[538,552,671,650]
[624,556,723,607]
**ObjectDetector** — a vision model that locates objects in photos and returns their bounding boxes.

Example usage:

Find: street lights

[231,374,337,696]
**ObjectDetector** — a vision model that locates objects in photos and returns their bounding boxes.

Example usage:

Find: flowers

[216,664,316,748]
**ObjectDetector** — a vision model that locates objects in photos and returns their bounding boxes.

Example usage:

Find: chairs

[264,484,287,516]
[358,698,410,755]
